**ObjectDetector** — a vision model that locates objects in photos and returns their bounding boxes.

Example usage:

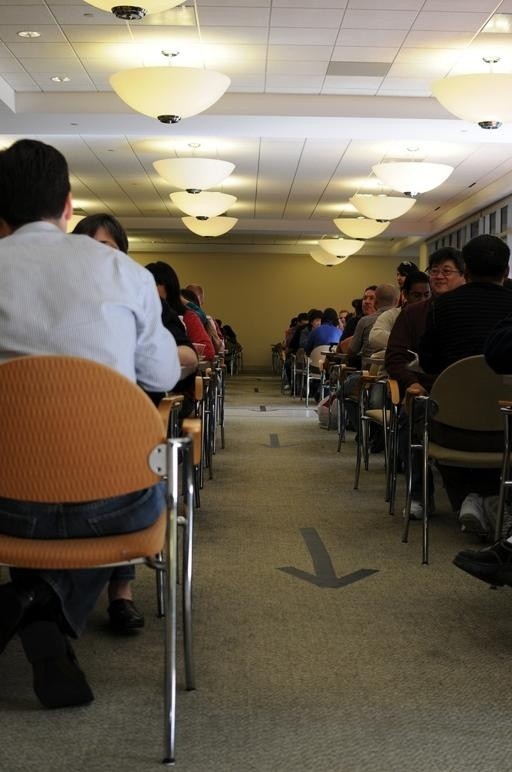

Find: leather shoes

[20,618,95,707]
[107,598,143,629]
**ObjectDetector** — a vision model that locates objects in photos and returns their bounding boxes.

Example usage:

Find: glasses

[428,266,460,277]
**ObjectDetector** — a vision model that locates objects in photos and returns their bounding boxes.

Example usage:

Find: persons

[0,139,180,707]
[286,260,430,452]
[386,246,465,521]
[420,234,512,542]
[66,214,196,632]
[144,261,225,359]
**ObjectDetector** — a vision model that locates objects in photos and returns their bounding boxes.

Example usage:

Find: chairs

[1,355,197,768]
[402,355,512,564]
[495,400,512,544]
[159,340,244,511]
[271,340,418,522]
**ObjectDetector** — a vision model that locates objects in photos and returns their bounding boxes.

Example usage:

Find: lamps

[82,0,184,19]
[155,158,236,191]
[108,66,229,124]
[318,239,365,258]
[351,194,416,223]
[180,216,238,239]
[433,73,512,128]
[333,218,390,241]
[169,190,237,221]
[311,248,347,267]
[372,163,454,197]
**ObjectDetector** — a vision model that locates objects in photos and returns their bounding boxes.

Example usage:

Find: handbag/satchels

[317,392,341,429]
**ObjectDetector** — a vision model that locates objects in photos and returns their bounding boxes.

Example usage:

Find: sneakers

[482,495,512,538]
[402,501,425,519]
[459,494,490,534]
[452,540,511,583]
